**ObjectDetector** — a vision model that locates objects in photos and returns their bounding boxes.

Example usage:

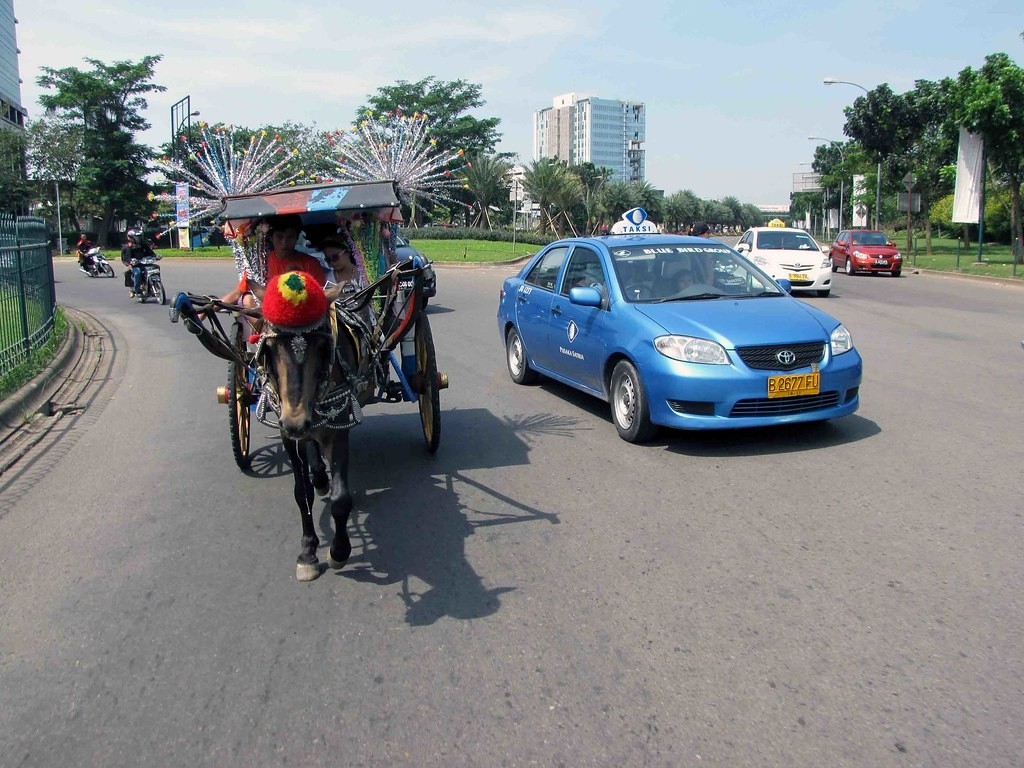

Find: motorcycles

[122,252,167,306]
[75,245,115,279]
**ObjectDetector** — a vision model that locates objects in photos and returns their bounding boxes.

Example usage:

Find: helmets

[127,229,143,244]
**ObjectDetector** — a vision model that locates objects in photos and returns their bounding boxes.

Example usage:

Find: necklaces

[335,264,355,284]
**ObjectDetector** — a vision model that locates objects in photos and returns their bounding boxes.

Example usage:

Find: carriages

[168,178,450,583]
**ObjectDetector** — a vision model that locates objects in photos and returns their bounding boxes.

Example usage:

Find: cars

[728,218,834,299]
[827,229,903,277]
[496,207,865,446]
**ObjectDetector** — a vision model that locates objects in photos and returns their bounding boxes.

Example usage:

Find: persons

[212,211,328,334]
[322,235,366,292]
[624,259,661,299]
[121,229,161,298]
[688,222,715,238]
[658,225,665,234]
[669,269,694,295]
[77,232,93,270]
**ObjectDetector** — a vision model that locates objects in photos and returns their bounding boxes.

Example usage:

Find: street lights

[797,161,831,244]
[51,180,63,258]
[175,112,201,183]
[824,79,881,231]
[808,136,845,232]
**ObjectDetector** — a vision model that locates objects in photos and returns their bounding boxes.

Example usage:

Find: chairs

[577,263,604,288]
[655,252,701,297]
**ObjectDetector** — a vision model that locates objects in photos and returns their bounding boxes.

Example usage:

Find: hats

[322,233,353,252]
[688,222,717,236]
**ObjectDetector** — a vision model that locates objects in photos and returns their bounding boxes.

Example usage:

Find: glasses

[324,248,348,264]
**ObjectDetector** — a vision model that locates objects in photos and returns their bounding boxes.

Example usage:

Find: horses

[244,277,365,582]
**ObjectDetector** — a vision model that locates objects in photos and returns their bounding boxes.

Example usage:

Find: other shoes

[136,294,143,302]
[129,290,135,298]
